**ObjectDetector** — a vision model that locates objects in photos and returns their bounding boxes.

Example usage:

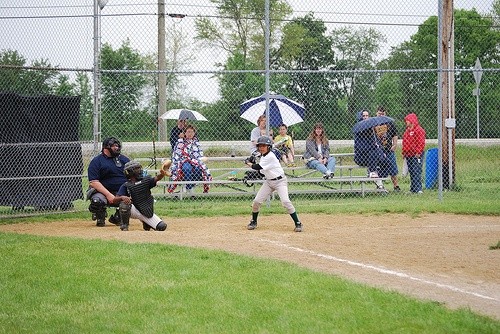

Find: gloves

[245,158,251,164]
[249,156,256,164]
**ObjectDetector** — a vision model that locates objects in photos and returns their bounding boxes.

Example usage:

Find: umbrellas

[239,94,306,128]
[160,107,208,133]
[352,116,393,146]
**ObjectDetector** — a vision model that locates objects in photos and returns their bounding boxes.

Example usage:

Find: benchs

[134,153,391,201]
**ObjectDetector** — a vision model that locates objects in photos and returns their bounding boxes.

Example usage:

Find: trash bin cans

[426,148,438,190]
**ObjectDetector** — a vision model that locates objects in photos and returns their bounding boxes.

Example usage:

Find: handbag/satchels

[402,158,408,178]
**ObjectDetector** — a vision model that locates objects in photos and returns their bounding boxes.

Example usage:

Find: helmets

[102,138,121,157]
[124,162,142,179]
[256,136,272,148]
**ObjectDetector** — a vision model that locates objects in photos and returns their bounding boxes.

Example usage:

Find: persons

[402,114,426,193]
[87,137,149,226]
[353,109,387,190]
[245,136,304,232]
[168,119,199,149]
[113,158,173,231]
[250,115,274,157]
[304,123,336,179]
[274,124,296,167]
[167,126,212,193]
[373,106,401,191]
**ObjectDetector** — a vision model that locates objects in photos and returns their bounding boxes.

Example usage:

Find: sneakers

[121,224,128,231]
[141,220,151,231]
[248,220,257,229]
[96,215,105,227]
[294,222,302,232]
[108,215,121,225]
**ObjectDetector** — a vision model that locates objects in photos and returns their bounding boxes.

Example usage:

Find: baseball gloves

[161,159,173,176]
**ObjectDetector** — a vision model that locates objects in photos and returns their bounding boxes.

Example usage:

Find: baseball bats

[242,138,288,165]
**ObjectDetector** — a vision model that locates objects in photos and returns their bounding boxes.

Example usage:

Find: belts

[272,176,282,180]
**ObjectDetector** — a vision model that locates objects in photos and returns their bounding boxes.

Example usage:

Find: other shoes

[327,172,334,178]
[377,185,385,189]
[394,186,401,190]
[418,190,423,193]
[323,175,328,179]
[288,163,296,167]
